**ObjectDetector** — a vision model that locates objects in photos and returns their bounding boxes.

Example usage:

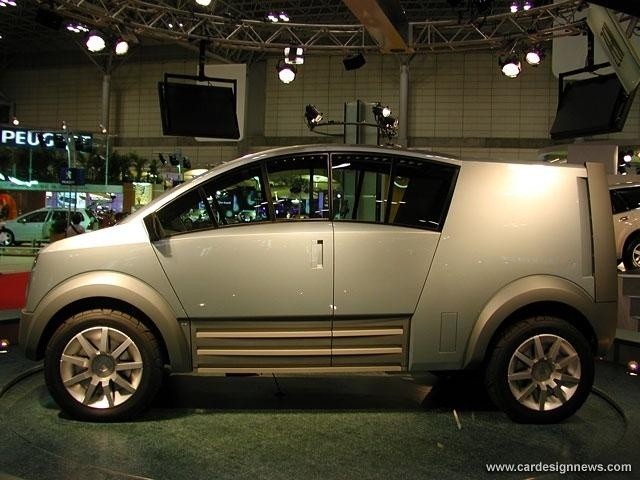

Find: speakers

[342,53,367,72]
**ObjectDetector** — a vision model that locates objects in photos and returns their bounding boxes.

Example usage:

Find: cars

[0,192,262,247]
[608,179,640,275]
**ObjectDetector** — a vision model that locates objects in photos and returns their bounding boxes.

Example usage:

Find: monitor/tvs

[550,73,637,140]
[158,81,240,140]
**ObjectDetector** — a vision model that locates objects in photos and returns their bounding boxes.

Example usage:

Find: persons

[49,218,69,244]
[68,213,86,237]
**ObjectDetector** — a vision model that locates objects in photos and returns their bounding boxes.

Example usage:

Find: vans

[16,138,615,424]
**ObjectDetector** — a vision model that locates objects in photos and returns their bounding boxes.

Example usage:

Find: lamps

[278,65,296,85]
[113,35,128,57]
[495,50,523,79]
[372,103,399,139]
[304,103,323,127]
[525,47,545,65]
[82,25,108,56]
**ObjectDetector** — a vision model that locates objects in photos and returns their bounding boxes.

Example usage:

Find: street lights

[9,116,20,181]
[98,122,109,183]
[62,121,71,169]
